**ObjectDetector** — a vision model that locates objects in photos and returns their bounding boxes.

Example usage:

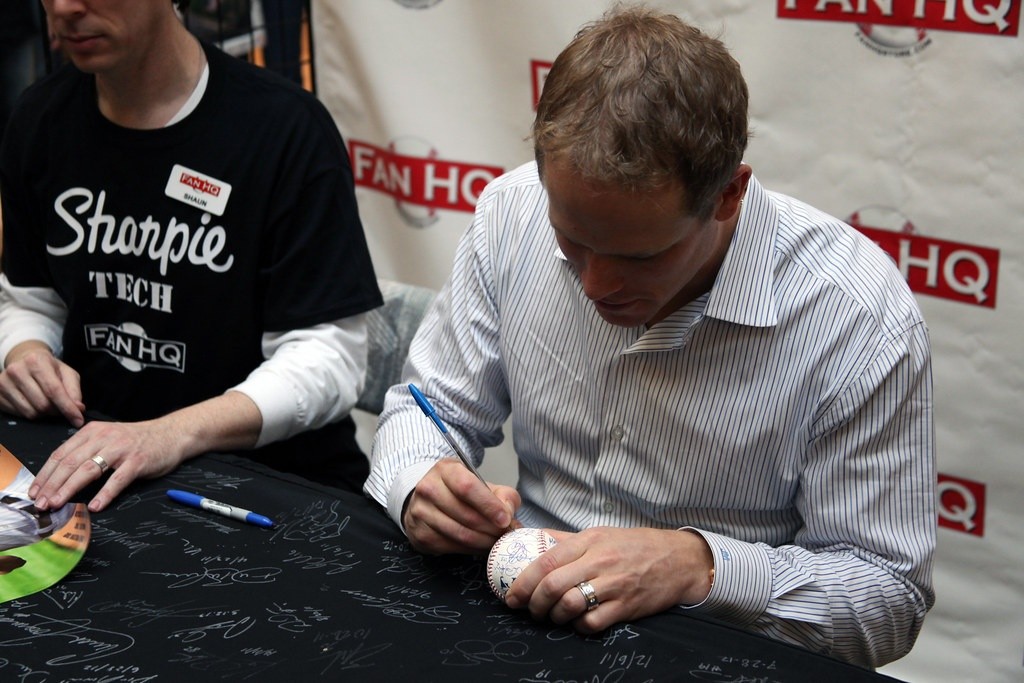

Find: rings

[572,581,600,612]
[90,454,110,475]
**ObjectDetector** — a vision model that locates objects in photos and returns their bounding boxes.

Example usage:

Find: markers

[167,489,277,529]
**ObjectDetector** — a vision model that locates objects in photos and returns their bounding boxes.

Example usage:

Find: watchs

[708,563,715,588]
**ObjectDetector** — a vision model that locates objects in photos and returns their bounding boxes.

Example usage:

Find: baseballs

[486,527,558,603]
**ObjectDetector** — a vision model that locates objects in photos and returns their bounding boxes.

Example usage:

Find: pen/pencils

[408,382,516,535]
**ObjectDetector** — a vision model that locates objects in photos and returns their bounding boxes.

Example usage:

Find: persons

[0,1,387,512]
[361,0,943,674]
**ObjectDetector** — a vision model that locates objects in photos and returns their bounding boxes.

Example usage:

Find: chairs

[344,277,449,423]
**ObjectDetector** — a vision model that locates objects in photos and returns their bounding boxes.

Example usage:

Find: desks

[1,369,906,681]
